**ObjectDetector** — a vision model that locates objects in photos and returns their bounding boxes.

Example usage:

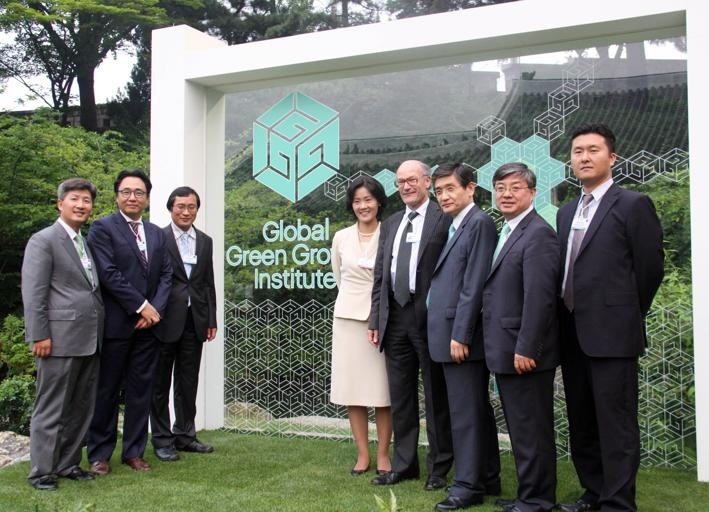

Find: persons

[553,123,666,511]
[328,177,404,476]
[422,163,497,511]
[479,163,566,511]
[87,172,176,474]
[18,176,104,491]
[367,159,456,490]
[150,187,218,460]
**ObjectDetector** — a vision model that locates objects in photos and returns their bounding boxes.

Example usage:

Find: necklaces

[358,231,375,237]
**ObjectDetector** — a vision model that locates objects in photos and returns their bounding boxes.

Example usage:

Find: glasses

[394,177,419,188]
[118,189,147,198]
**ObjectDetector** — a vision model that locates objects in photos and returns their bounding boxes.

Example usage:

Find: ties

[131,223,146,267]
[394,212,418,308]
[564,195,593,313]
[448,224,455,242]
[492,224,511,268]
[75,235,90,279]
[181,234,194,279]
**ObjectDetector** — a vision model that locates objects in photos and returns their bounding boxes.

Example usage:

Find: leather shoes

[34,439,213,490]
[351,466,591,512]
